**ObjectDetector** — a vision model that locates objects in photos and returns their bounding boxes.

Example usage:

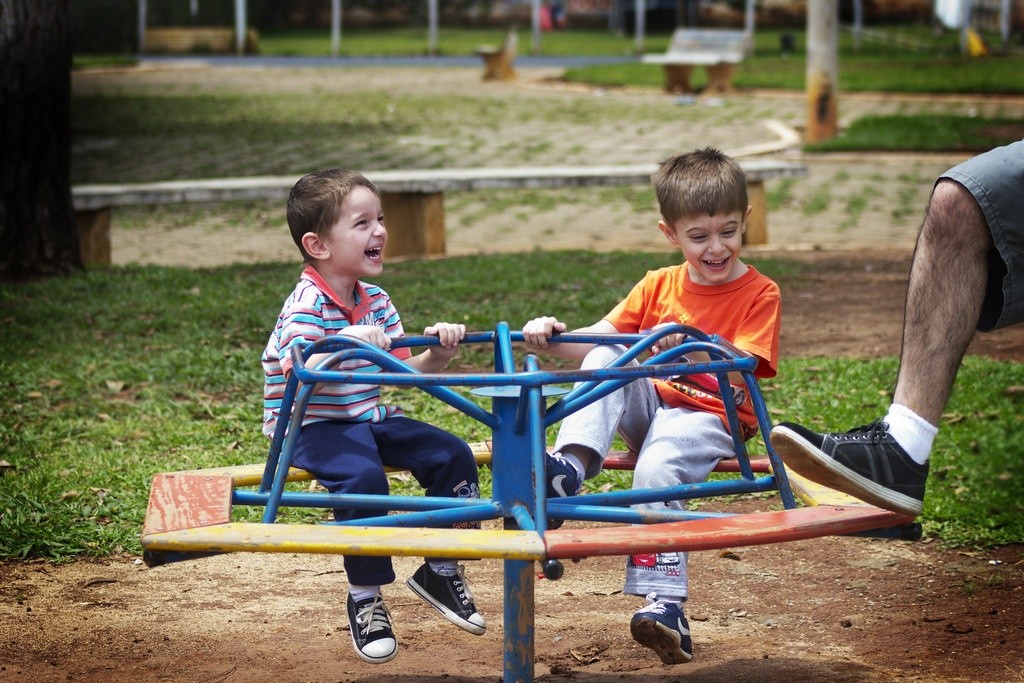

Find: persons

[523,147,781,666]
[262,168,487,664]
[769,138,1024,515]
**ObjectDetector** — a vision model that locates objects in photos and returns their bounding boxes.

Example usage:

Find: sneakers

[406,561,489,635]
[346,592,399,663]
[625,592,694,667]
[769,418,931,518]
[542,452,582,498]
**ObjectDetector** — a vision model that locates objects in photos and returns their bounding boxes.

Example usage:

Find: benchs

[472,22,519,82]
[71,154,812,269]
[640,27,754,98]
